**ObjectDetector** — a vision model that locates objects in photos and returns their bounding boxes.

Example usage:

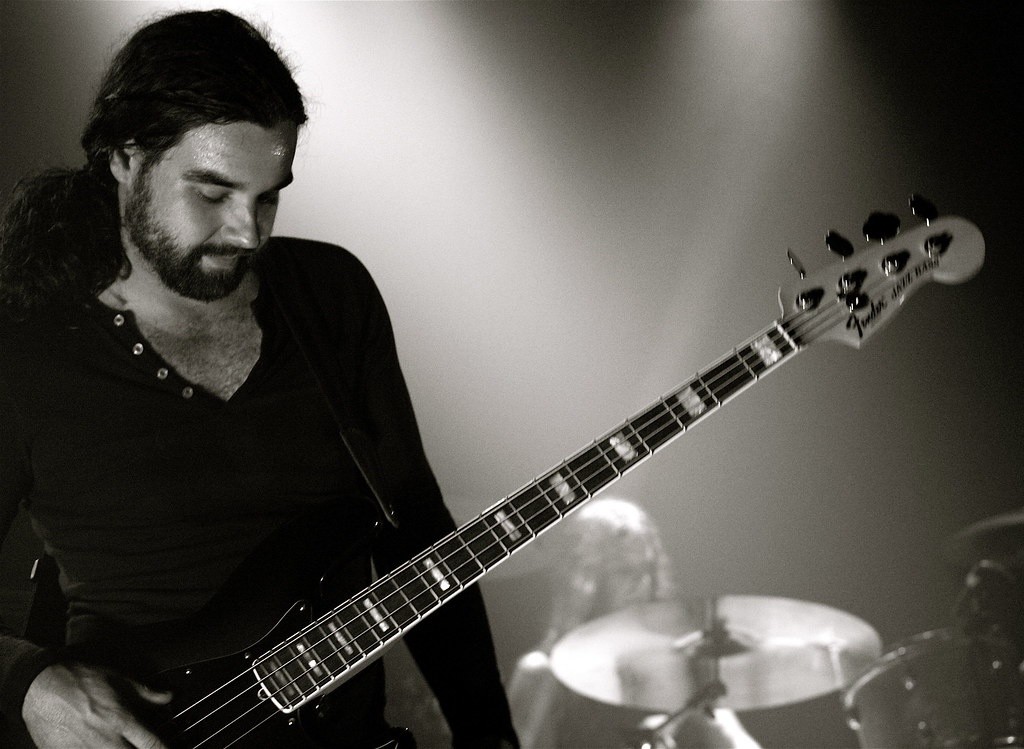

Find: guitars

[116,189,987,749]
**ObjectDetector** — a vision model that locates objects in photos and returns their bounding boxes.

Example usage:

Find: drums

[842,612,1024,749]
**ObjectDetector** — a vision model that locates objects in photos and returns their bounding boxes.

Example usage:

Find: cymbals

[548,589,885,713]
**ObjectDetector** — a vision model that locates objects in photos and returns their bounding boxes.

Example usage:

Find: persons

[509,498,764,749]
[0,10,522,749]
